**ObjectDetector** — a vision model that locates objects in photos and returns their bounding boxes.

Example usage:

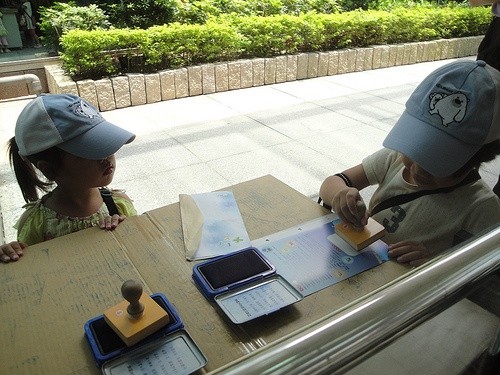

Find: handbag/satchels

[30,16,36,25]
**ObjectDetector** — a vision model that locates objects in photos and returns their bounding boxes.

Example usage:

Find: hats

[382,60,500,178]
[14,94,136,160]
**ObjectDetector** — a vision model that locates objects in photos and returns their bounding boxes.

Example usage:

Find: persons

[0,12,8,53]
[0,93,136,264]
[470,0,500,197]
[318,60,500,268]
[12,0,42,49]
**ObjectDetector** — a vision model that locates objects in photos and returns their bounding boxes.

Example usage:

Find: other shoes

[5,49,11,53]
[0,50,3,54]
[32,44,42,49]
